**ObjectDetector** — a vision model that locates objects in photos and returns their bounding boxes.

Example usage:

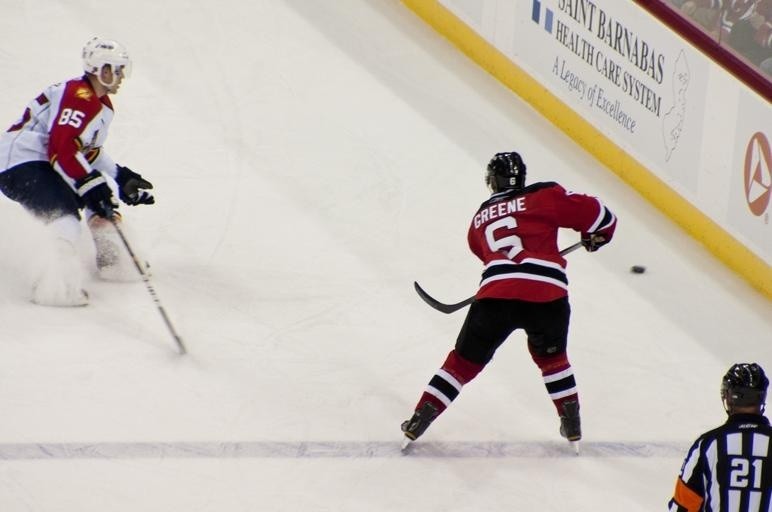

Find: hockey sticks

[111,217,186,355]
[414,242,585,314]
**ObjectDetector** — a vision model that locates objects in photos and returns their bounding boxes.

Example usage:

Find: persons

[0,37,155,306]
[667,362,772,512]
[671,0,772,79]
[385,150,617,453]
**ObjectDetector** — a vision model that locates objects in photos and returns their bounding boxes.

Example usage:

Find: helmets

[82,34,129,77]
[484,152,526,186]
[720,361,770,406]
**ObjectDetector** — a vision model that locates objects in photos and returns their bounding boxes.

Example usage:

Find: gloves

[580,215,616,252]
[73,169,118,220]
[115,164,154,206]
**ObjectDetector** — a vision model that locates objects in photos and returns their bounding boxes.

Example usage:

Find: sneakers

[94,245,151,273]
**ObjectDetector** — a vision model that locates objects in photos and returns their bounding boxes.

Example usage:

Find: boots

[557,399,582,444]
[399,401,436,441]
